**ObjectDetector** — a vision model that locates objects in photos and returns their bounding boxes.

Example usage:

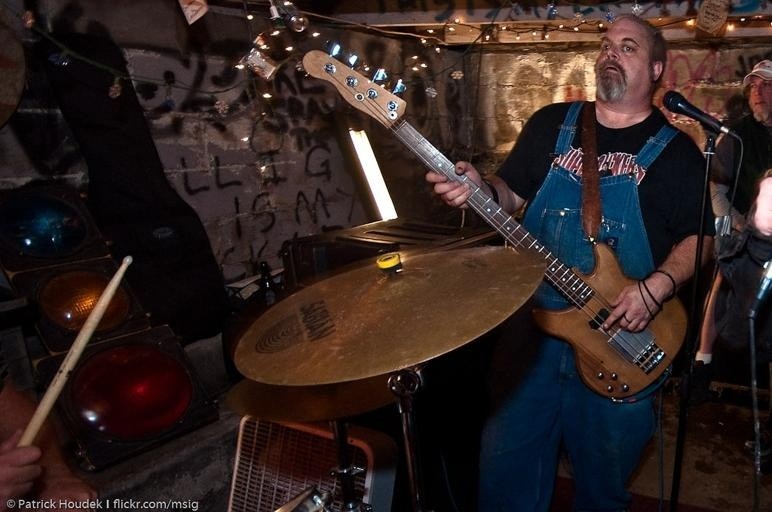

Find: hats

[744,60,772,87]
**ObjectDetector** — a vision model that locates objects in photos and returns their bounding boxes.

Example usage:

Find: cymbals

[225,375,396,422]
[235,246,547,386]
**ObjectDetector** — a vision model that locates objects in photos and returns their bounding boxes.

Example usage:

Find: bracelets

[642,278,664,313]
[637,279,655,320]
[647,270,677,304]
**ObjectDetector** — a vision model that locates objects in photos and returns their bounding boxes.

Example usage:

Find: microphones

[661,88,735,140]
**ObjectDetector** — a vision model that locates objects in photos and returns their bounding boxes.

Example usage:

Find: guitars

[295,41,688,404]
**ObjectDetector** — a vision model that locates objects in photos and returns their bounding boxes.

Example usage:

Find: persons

[425,10,719,510]
[741,167,771,266]
[672,59,771,407]
[1,371,104,511]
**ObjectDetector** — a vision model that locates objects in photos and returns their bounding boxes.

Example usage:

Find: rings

[621,314,634,325]
